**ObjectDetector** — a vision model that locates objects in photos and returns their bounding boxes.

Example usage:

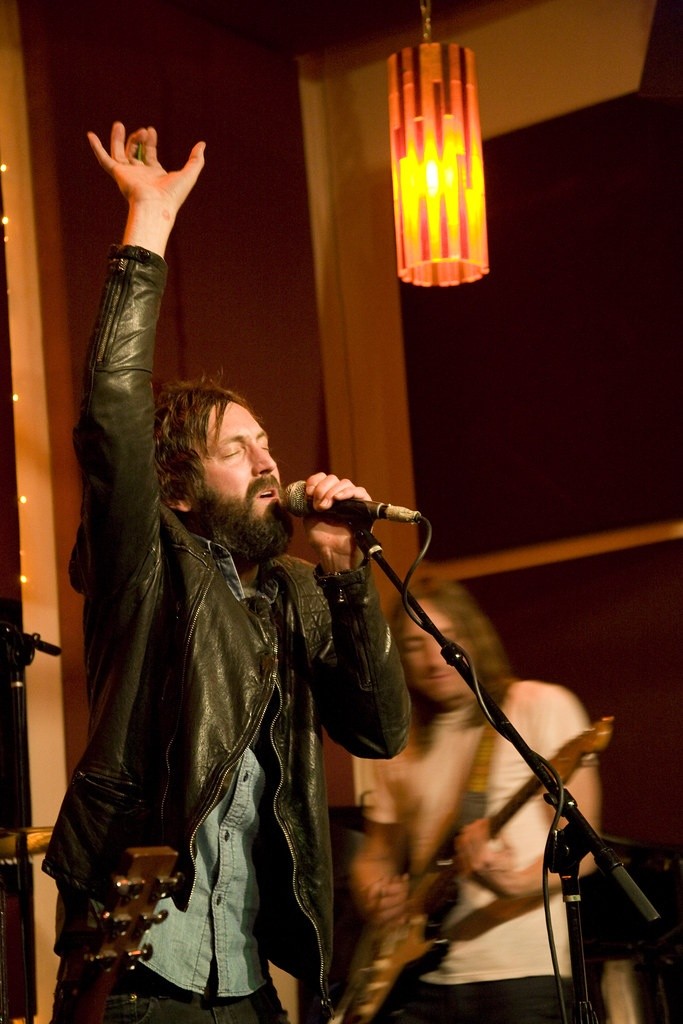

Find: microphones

[284,480,422,524]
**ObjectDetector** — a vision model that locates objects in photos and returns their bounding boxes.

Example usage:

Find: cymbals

[1,827,54,864]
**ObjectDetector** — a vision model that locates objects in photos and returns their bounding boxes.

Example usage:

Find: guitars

[350,712,615,1019]
[74,846,186,1023]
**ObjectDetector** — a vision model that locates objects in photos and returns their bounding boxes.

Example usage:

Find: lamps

[383,0,488,287]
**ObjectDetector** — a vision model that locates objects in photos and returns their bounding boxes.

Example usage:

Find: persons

[349,583,600,1023]
[40,119,412,1024]
